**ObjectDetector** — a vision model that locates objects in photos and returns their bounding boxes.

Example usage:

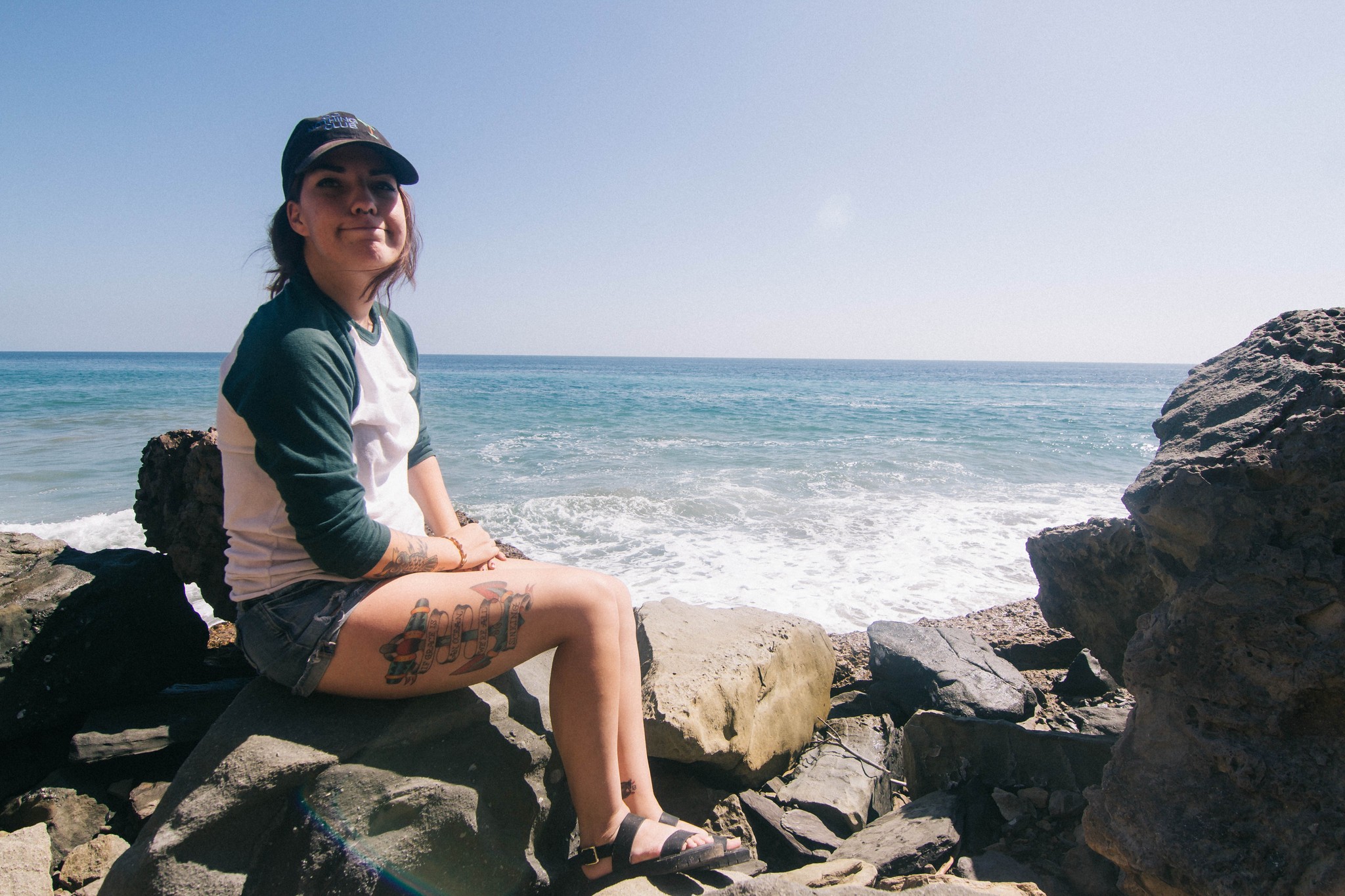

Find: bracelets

[438,535,466,571]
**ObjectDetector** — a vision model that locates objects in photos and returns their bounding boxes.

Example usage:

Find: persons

[217,111,751,890]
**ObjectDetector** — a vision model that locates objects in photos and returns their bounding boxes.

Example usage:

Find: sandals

[566,812,724,890]
[658,810,750,873]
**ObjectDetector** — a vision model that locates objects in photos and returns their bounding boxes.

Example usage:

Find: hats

[281,111,419,199]
